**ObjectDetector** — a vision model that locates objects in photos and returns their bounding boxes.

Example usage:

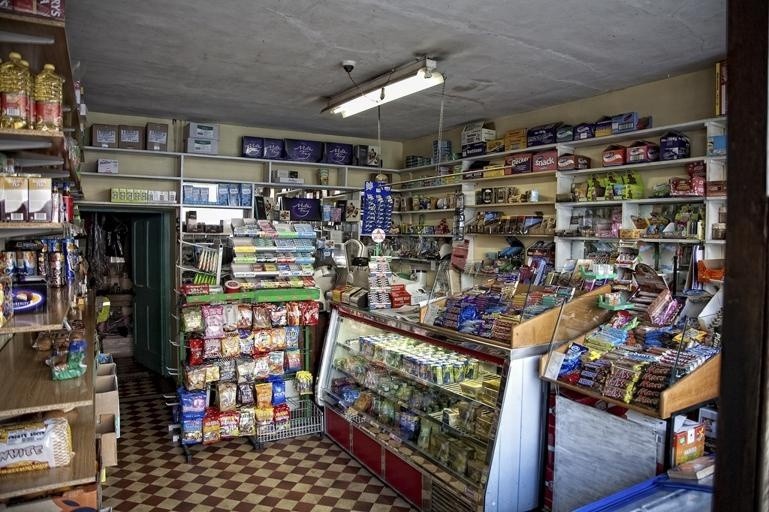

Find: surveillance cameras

[341,60,357,72]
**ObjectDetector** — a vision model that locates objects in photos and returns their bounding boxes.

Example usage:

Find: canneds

[359,332,479,385]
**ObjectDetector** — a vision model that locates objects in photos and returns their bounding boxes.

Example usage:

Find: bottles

[399,388,448,450]
[51,180,74,223]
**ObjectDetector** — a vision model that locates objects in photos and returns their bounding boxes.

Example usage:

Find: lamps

[319,56,447,120]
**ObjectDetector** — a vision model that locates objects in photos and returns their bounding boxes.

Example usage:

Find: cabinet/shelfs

[396,118,727,278]
[80,145,396,259]
[1,9,98,504]
